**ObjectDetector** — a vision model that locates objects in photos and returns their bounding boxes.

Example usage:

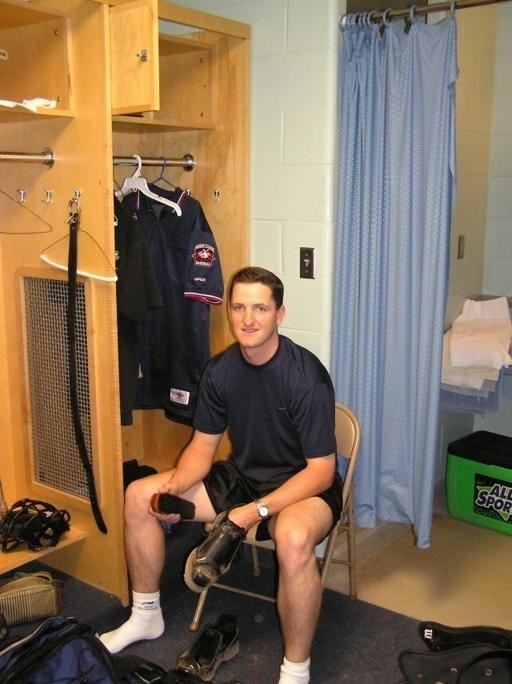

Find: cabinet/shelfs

[0,3,253,603]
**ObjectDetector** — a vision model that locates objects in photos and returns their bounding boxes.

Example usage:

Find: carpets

[2,530,511,684]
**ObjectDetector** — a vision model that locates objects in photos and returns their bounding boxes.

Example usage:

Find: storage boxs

[447,430,511,538]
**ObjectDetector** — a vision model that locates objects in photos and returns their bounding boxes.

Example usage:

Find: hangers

[1,153,182,285]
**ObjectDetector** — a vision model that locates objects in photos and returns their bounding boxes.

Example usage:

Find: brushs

[151,493,196,520]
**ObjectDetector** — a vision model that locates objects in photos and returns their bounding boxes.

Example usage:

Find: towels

[432,294,512,396]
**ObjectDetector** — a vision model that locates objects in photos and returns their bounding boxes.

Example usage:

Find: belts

[62,193,111,537]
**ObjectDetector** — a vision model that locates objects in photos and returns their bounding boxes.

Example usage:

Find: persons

[96,264,345,684]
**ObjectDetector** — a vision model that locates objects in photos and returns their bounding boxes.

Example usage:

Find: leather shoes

[174,612,244,682]
[182,500,251,594]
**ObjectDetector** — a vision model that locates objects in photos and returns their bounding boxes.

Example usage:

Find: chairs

[187,402,359,634]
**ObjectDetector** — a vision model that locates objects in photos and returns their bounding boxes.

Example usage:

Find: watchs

[253,496,270,522]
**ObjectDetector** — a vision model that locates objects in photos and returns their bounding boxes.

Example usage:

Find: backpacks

[0,612,141,684]
[0,568,63,628]
